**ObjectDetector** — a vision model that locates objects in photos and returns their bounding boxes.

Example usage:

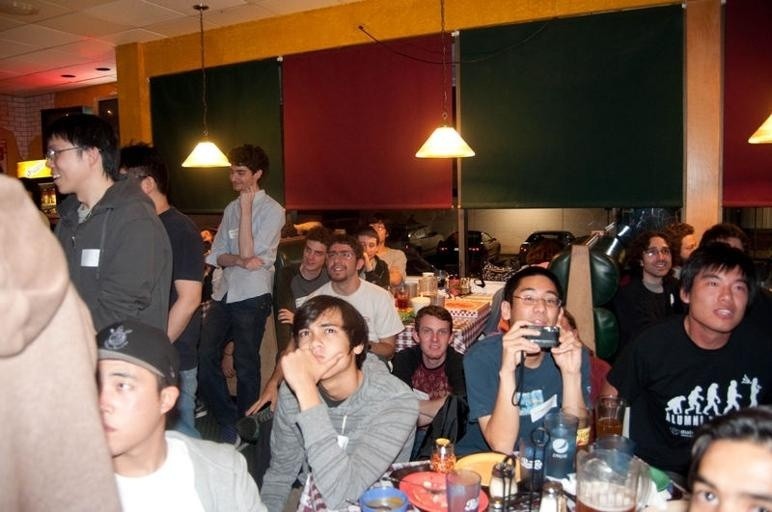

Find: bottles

[39,186,56,205]
[429,437,455,475]
[488,454,519,500]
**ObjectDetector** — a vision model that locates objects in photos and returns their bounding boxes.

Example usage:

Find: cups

[446,468,482,512]
[594,397,626,439]
[395,269,459,316]
[544,409,579,477]
[596,434,634,467]
[560,405,592,451]
[516,435,546,490]
[544,452,651,512]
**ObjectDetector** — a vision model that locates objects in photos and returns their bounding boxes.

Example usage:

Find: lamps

[747,114,772,146]
[180,4,232,171]
[413,0,477,162]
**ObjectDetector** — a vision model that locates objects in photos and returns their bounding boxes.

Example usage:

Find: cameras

[523,324,561,349]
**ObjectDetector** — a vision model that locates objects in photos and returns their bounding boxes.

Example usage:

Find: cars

[389,224,443,262]
[437,230,501,270]
[519,230,575,269]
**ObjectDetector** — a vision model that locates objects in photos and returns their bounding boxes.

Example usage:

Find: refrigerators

[15,160,63,230]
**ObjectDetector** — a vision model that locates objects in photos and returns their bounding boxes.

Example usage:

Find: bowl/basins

[358,486,408,512]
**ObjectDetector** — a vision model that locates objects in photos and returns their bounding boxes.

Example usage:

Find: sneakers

[223,405,275,453]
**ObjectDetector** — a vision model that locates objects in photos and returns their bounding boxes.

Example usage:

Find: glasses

[45,146,76,159]
[512,295,562,308]
[643,249,670,256]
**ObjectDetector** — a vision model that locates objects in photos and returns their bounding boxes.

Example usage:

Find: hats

[97,320,180,385]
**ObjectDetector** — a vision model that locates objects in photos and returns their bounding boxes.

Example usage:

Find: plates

[454,452,528,487]
[399,471,490,512]
[645,464,671,495]
